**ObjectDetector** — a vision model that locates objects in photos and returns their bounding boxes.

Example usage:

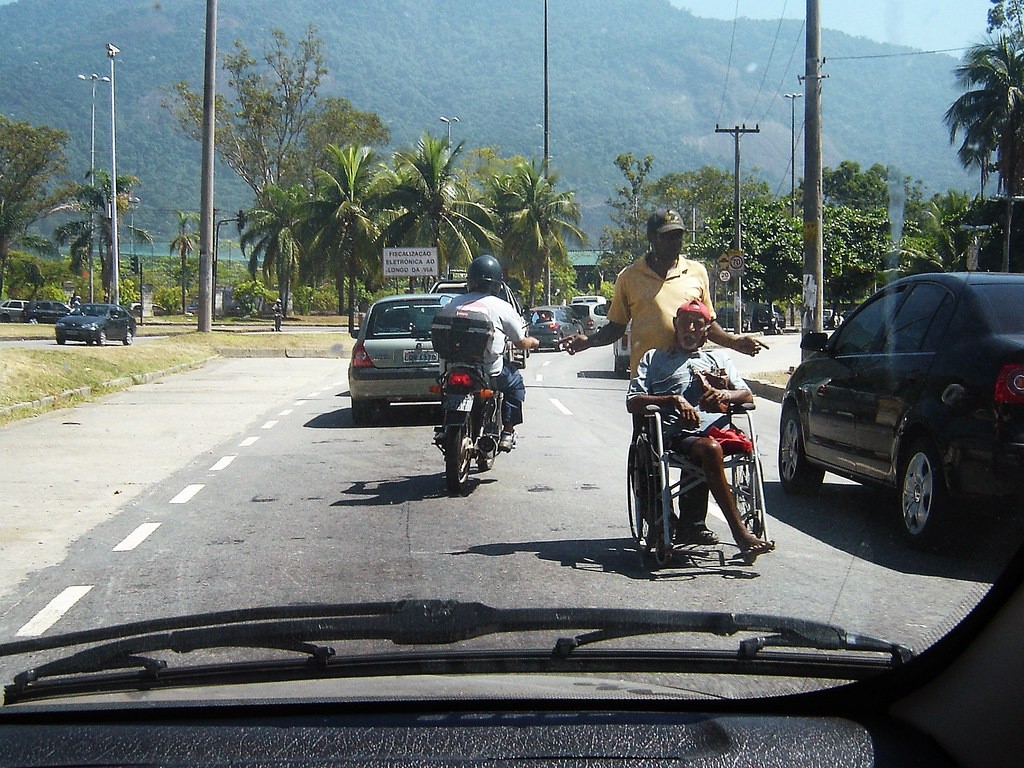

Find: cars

[523,305,584,350]
[715,307,750,331]
[54,303,137,348]
[347,294,464,420]
[822,308,844,328]
[778,268,1023,544]
[567,302,611,334]
[0,300,30,323]
[22,301,72,325]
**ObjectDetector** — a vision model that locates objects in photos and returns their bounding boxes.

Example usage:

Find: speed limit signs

[721,270,735,283]
[729,254,745,272]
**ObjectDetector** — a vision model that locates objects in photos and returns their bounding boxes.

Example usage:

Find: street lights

[783,93,804,218]
[990,192,1024,274]
[105,42,122,303]
[77,71,110,304]
[128,197,141,272]
[440,116,460,160]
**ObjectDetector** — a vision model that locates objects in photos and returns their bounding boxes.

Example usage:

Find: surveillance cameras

[105,43,121,53]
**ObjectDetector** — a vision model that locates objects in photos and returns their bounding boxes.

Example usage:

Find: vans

[572,296,608,305]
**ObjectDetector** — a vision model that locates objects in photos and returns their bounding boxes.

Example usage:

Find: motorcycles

[434,293,539,493]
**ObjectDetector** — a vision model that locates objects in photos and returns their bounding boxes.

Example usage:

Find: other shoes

[434,429,446,442]
[644,509,677,549]
[674,518,719,546]
[498,430,516,451]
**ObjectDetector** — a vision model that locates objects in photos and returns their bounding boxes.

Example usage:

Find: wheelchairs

[628,399,778,572]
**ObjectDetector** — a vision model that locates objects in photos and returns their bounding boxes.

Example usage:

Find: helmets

[466,255,502,294]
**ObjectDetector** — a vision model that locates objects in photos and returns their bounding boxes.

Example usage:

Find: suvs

[419,279,531,368]
[750,303,789,330]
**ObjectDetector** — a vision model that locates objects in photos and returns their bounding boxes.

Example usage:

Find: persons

[272,299,284,331]
[625,296,772,565]
[435,255,540,451]
[554,208,771,544]
[74,296,82,305]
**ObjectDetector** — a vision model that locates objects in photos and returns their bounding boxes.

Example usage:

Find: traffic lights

[130,255,140,275]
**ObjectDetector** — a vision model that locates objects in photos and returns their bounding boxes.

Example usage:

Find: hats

[647,208,686,234]
[675,299,711,324]
